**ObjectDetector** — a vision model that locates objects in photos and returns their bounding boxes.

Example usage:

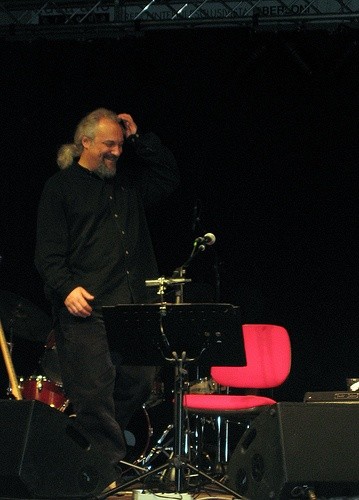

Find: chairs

[170,323,293,461]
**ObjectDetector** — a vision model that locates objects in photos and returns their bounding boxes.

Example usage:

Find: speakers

[225,401,359,500]
[0,397,123,500]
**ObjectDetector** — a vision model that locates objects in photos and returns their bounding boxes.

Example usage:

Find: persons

[29,109,182,500]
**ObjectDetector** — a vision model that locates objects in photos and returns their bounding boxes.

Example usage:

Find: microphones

[144,278,192,287]
[195,232,216,245]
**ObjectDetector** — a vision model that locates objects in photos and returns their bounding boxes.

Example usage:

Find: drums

[9,375,71,412]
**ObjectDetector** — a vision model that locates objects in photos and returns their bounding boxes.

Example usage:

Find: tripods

[94,302,242,500]
